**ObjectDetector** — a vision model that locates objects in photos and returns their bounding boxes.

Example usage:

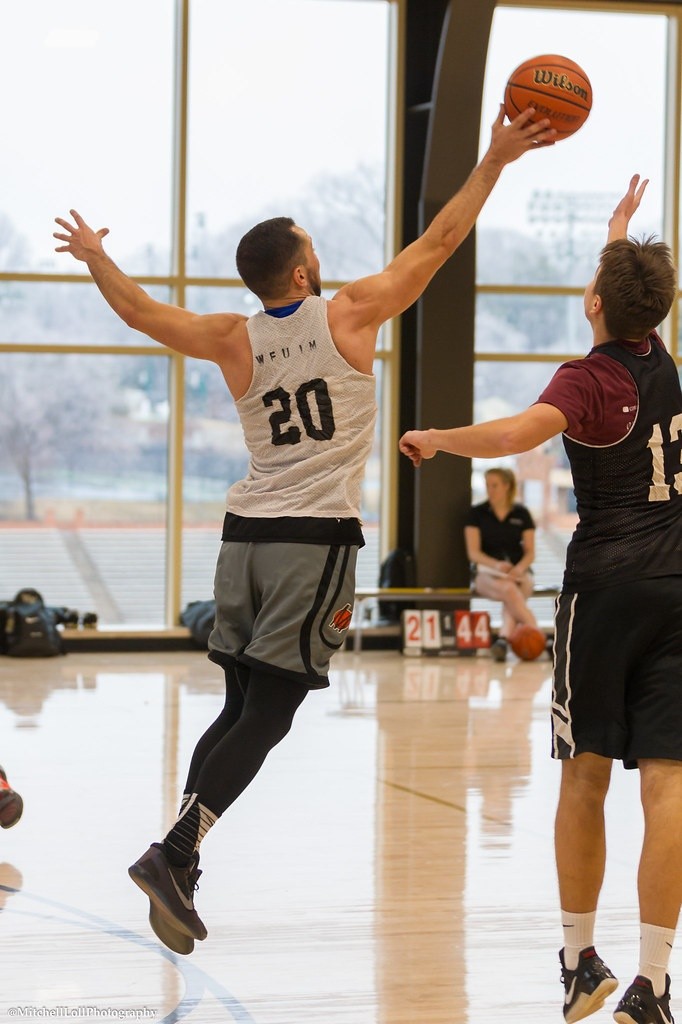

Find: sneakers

[490,638,508,662]
[559,946,618,1024]
[545,636,554,663]
[128,842,209,956]
[614,972,677,1024]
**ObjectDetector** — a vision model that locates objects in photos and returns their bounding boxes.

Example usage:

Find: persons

[465,469,553,662]
[53,102,558,956]
[398,174,681,1024]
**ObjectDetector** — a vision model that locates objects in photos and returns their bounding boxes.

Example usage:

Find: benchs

[349,587,561,664]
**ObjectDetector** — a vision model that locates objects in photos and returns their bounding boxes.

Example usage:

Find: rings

[533,140,540,145]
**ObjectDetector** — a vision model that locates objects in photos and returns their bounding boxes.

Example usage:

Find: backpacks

[0,588,62,658]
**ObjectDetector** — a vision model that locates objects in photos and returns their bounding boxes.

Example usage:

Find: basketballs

[509,626,546,662]
[503,53,594,143]
